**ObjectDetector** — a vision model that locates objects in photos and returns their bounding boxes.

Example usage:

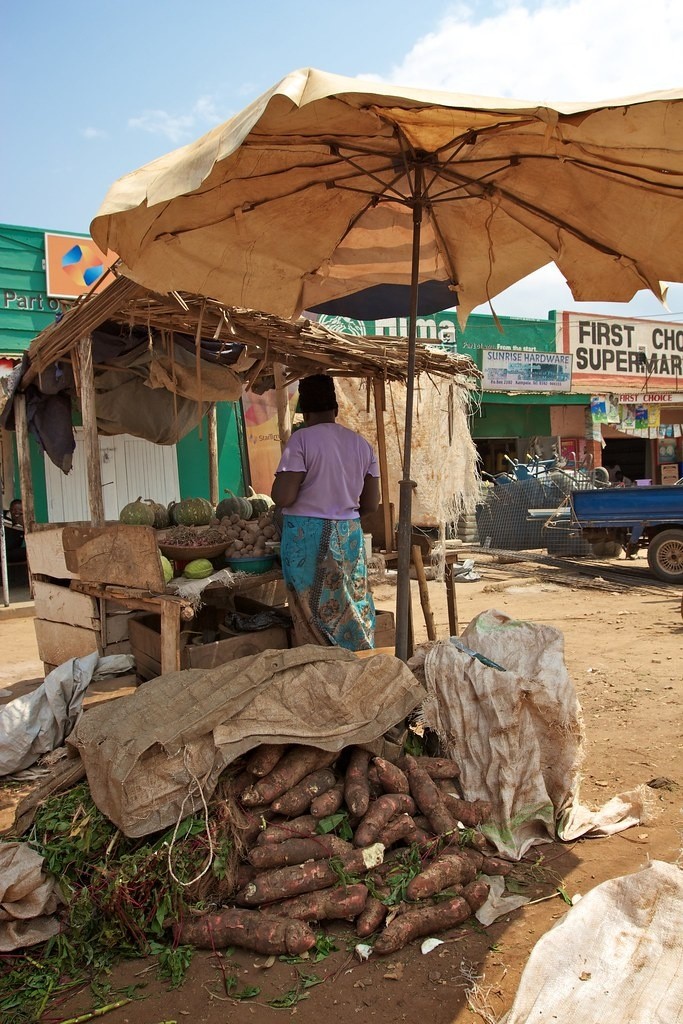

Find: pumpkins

[120,486,275,528]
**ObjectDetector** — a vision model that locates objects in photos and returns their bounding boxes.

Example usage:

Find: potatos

[177,742,512,956]
[210,510,280,558]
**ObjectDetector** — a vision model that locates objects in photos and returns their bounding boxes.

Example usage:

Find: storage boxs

[280,607,397,649]
[128,595,288,681]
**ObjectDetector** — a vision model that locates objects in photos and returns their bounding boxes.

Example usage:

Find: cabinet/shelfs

[658,464,678,485]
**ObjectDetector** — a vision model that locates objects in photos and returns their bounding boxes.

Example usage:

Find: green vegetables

[0,780,490,1024]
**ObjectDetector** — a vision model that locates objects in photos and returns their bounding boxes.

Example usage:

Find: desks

[70,550,471,676]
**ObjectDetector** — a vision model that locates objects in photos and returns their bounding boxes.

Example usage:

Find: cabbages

[160,556,173,584]
[184,558,213,579]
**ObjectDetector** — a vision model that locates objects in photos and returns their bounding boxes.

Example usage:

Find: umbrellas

[90,67,683,662]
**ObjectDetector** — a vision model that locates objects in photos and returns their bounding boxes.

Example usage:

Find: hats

[295,374,338,413]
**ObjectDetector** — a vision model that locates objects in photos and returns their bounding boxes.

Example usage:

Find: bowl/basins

[156,540,234,559]
[225,555,275,574]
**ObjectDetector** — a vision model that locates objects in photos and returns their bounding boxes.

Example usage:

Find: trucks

[571,485,683,584]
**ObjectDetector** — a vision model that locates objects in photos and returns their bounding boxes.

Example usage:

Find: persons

[274,374,380,651]
[615,471,632,484]
[4,499,23,527]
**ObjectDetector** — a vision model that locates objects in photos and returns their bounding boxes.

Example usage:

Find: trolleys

[476,452,622,557]
